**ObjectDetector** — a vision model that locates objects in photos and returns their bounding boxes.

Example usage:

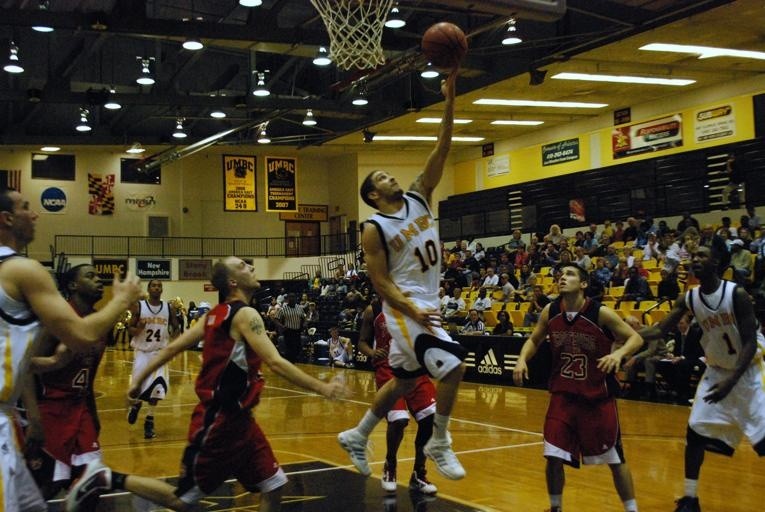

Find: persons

[67,255,345,511]
[513,264,645,510]
[355,293,439,495]
[130,280,178,441]
[109,298,214,351]
[440,204,765,405]
[21,263,125,510]
[0,186,148,512]
[260,249,377,369]
[640,244,765,511]
[336,62,467,479]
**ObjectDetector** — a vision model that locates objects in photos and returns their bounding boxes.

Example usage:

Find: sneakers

[423,432,465,480]
[143,416,155,439]
[66,459,112,512]
[337,428,373,476]
[409,470,438,494]
[127,402,142,424]
[673,496,701,512]
[382,458,397,492]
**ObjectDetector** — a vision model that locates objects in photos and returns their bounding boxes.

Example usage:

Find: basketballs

[422,21,468,68]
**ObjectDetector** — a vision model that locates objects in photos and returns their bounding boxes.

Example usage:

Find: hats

[729,239,744,246]
[501,274,509,279]
